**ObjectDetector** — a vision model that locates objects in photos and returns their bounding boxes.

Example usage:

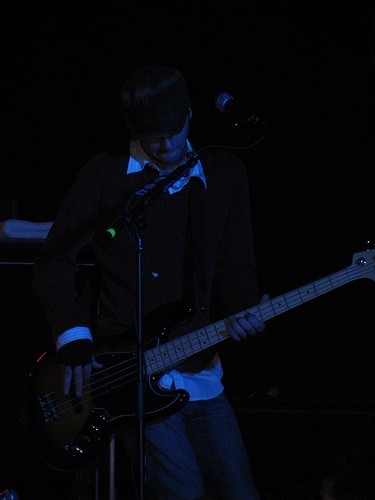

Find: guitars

[19,240,375,473]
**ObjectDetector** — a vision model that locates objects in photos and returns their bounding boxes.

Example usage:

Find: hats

[119,64,192,143]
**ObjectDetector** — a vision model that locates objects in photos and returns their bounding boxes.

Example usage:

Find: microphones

[0,489,20,500]
[215,92,264,126]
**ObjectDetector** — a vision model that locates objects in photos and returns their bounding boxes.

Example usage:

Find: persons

[33,63,270,500]
